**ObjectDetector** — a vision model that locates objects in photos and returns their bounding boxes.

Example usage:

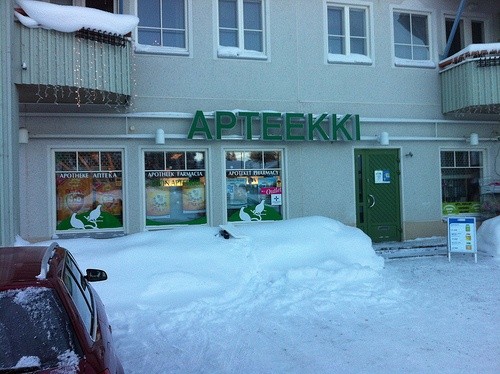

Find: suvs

[0,242,125,374]
